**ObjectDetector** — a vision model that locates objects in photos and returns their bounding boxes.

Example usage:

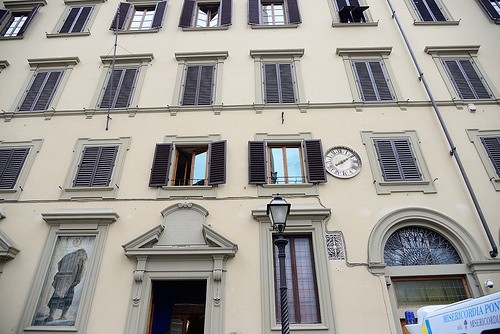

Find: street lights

[266,195,291,334]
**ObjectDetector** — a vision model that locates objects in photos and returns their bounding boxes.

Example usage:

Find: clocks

[324,146,362,179]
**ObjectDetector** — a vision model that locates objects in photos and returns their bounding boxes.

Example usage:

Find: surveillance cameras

[487,281,494,288]
